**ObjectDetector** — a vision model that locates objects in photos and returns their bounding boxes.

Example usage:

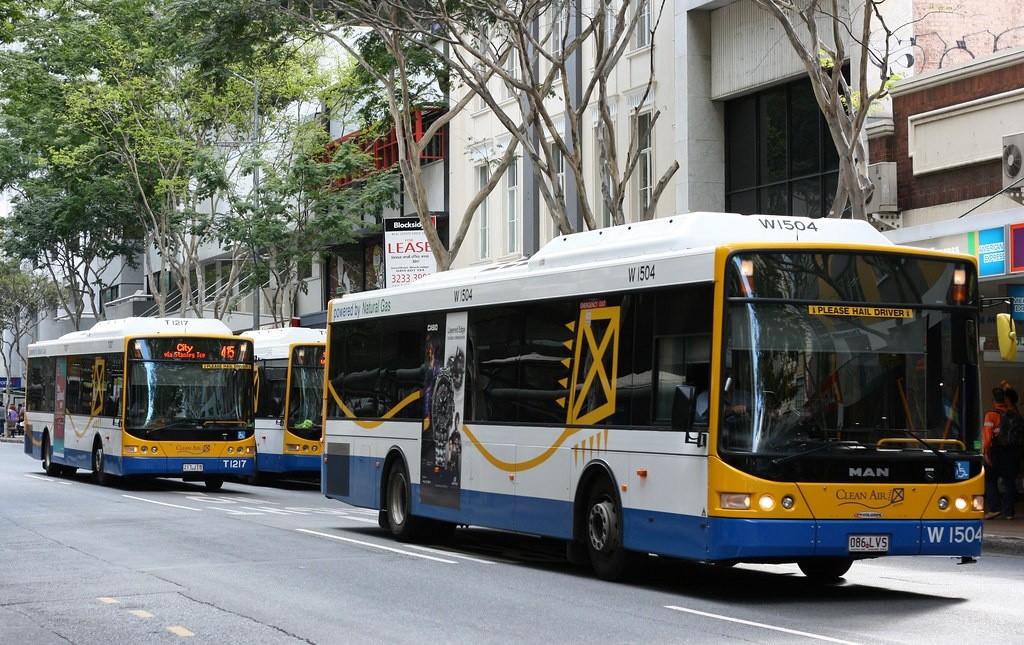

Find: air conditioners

[864,162,897,213]
[1002,132,1024,190]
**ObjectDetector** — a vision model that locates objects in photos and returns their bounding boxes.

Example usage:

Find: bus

[238,325,327,485]
[318,211,1019,581]
[22,315,268,492]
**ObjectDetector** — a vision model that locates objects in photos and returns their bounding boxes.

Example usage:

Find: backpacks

[988,407,1024,460]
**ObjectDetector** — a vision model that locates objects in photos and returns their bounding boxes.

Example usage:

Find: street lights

[215,64,262,330]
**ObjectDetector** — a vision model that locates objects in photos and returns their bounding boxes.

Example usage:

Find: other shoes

[1005,513,1014,519]
[984,512,1001,519]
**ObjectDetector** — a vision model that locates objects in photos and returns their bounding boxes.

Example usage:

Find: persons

[691,369,751,442]
[0,401,25,439]
[980,380,1024,521]
